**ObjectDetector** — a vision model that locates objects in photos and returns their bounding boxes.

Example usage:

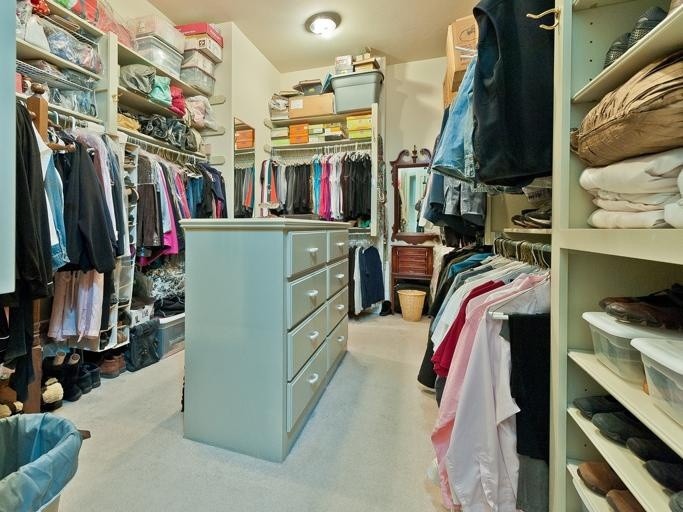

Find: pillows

[567,49,683,168]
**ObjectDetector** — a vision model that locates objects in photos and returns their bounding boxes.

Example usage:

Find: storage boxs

[153,311,186,360]
[442,14,477,109]
[235,128,254,150]
[580,310,683,428]
[271,52,386,148]
[176,22,224,96]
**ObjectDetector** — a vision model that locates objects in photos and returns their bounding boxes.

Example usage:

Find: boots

[380,301,391,316]
[64,352,126,401]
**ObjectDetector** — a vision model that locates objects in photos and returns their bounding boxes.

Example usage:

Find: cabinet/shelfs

[546,0,683,512]
[66,131,141,354]
[391,245,434,316]
[14,0,211,156]
[178,216,354,463]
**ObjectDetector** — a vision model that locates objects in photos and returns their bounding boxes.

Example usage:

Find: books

[299,80,322,85]
[279,90,301,95]
[351,58,380,72]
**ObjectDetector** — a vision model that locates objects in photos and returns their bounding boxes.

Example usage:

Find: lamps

[305,10,343,36]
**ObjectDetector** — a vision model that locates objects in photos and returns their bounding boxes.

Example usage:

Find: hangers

[351,232,376,253]
[267,140,372,167]
[485,236,553,321]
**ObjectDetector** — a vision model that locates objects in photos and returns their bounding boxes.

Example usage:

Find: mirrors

[233,116,256,217]
[387,145,441,245]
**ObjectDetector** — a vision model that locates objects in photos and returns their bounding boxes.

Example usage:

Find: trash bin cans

[0,413,90,511]
[396,289,426,321]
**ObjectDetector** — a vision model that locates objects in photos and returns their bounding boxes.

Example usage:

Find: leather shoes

[598,283,682,327]
[573,395,682,511]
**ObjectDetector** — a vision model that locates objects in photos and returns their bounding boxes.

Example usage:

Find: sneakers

[511,208,551,228]
[604,6,667,68]
